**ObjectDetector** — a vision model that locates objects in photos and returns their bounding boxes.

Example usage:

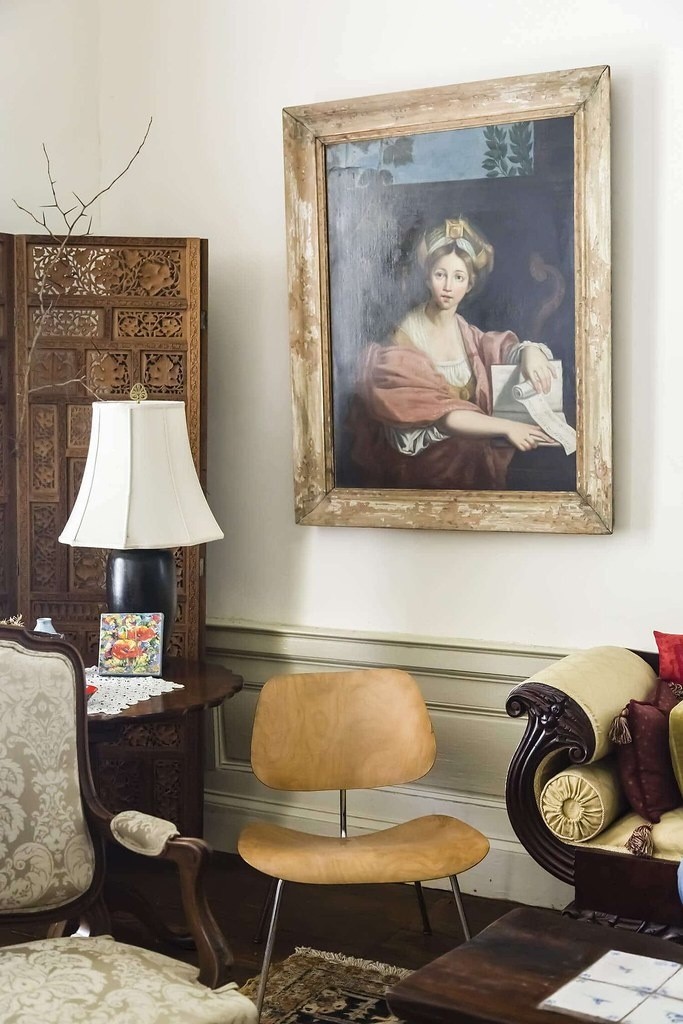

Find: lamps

[59,382,224,655]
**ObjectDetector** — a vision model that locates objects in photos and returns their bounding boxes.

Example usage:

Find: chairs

[0,626,262,1024]
[236,669,490,1024]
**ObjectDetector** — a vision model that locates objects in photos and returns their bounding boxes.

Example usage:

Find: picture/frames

[282,64,614,535]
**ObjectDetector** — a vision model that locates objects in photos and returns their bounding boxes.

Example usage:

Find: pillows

[653,631,683,685]
[538,766,630,844]
[669,700,683,796]
[607,682,683,858]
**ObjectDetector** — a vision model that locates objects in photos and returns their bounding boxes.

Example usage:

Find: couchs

[504,630,683,944]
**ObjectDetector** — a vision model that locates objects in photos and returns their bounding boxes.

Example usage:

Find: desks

[41,656,242,988]
[385,908,683,1024]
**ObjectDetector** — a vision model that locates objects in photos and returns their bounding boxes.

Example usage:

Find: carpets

[236,946,417,1024]
